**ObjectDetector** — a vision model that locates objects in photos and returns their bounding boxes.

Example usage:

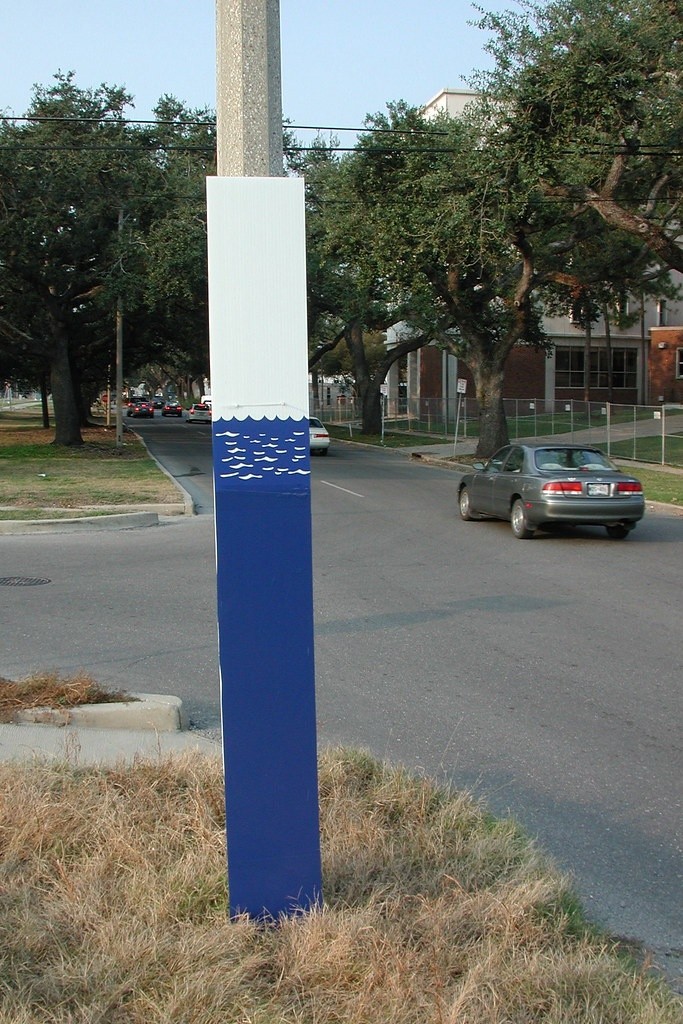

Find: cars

[185,404,212,423]
[161,401,182,417]
[126,402,154,418]
[309,417,330,456]
[456,442,644,540]
